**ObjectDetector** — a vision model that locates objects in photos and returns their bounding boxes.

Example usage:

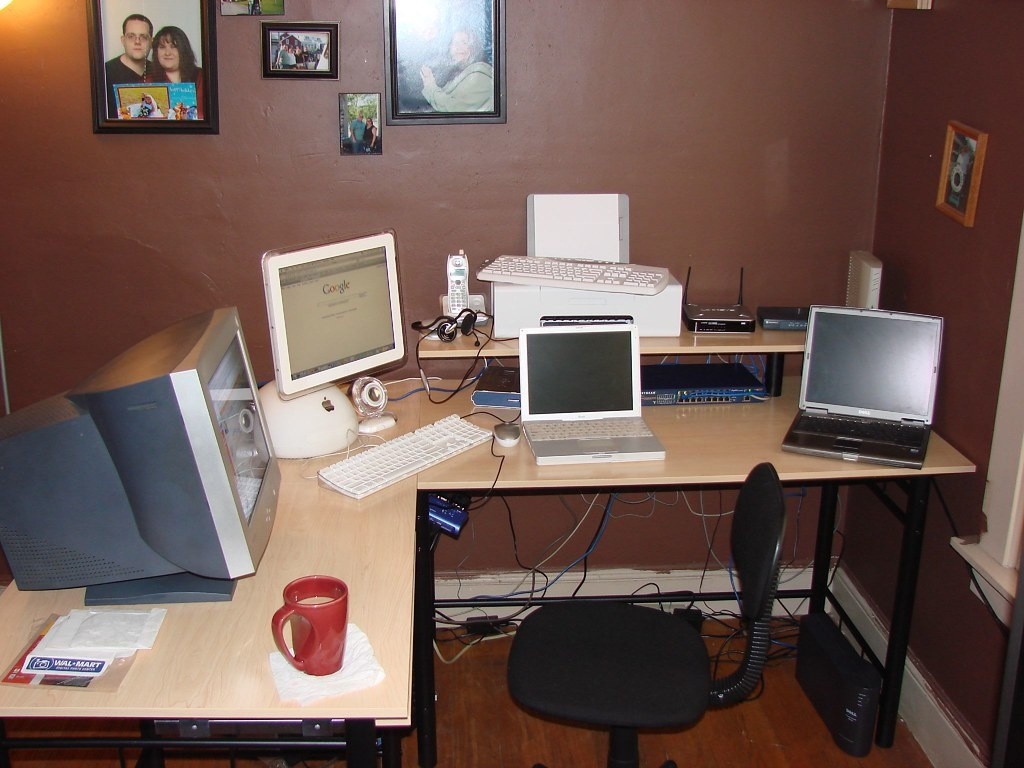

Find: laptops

[782,304,944,469]
[520,324,666,466]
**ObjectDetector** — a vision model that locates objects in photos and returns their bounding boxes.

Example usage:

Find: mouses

[493,421,520,448]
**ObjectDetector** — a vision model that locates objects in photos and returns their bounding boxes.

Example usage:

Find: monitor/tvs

[259,226,408,460]
[0,306,282,606]
[207,336,243,418]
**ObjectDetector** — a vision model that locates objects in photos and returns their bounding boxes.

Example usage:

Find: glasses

[125,32,152,43]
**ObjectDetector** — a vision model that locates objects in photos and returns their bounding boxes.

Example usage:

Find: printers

[490,193,683,340]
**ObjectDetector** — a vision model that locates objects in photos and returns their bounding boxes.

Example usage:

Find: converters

[466,616,499,634]
[673,608,703,633]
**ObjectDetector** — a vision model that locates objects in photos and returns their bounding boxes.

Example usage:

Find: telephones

[440,249,489,328]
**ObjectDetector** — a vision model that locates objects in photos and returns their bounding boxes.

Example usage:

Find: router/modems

[682,266,756,333]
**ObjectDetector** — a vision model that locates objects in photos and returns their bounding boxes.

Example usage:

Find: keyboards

[233,476,262,523]
[476,253,669,295]
[318,414,493,500]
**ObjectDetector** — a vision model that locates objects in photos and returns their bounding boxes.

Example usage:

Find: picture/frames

[928,118,989,228]
[382,0,507,124]
[259,18,343,81]
[337,92,383,156]
[84,0,221,135]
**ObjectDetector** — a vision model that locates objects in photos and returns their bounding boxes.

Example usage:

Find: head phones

[411,308,477,342]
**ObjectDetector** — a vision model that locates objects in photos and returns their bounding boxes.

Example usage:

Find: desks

[0,327,980,768]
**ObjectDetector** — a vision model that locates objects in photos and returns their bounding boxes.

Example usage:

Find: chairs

[508,461,790,768]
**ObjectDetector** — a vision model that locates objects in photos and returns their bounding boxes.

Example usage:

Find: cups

[272,574,348,675]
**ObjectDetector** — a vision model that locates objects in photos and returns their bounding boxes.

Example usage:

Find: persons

[420,25,494,111]
[273,44,328,70]
[351,113,377,154]
[145,26,204,120]
[105,14,153,119]
